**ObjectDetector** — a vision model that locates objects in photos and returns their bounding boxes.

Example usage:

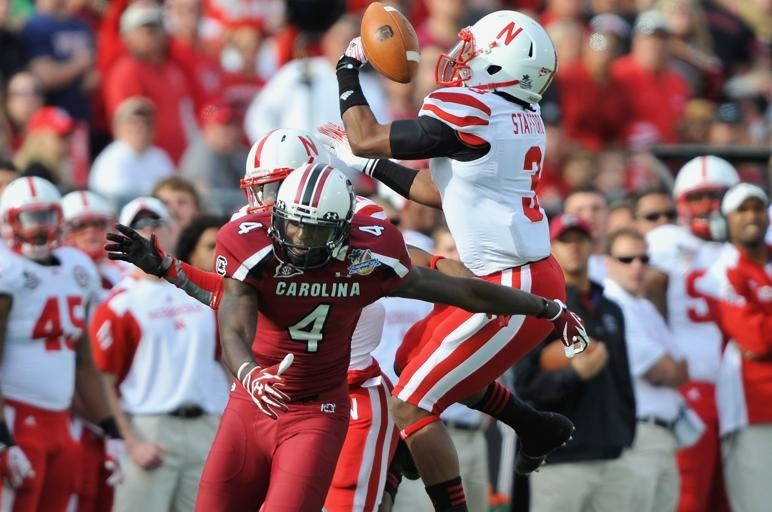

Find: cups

[646,211,676,222]
[618,255,651,265]
[131,215,166,230]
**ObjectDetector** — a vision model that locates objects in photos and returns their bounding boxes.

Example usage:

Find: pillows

[63,191,118,263]
[268,161,359,271]
[433,10,557,107]
[672,155,742,239]
[239,128,320,226]
[1,177,66,263]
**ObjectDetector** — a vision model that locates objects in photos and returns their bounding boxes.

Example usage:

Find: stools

[168,408,203,419]
[639,416,670,428]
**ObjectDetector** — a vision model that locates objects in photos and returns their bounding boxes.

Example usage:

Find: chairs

[513,414,576,476]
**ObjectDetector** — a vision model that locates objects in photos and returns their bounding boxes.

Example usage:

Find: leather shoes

[102,437,130,486]
[319,121,382,182]
[336,37,367,72]
[547,297,591,358]
[105,224,173,282]
[237,353,295,420]
[0,444,36,490]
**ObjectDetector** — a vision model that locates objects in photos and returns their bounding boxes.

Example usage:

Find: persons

[195,165,589,512]
[103,128,506,512]
[1,0,772,512]
[336,10,567,512]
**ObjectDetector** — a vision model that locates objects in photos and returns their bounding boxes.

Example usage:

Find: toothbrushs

[361,1,421,83]
[539,337,605,373]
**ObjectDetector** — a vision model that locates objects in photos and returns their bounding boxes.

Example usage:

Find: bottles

[117,195,172,231]
[549,212,593,242]
[119,0,163,31]
[27,106,75,137]
[720,181,769,216]
